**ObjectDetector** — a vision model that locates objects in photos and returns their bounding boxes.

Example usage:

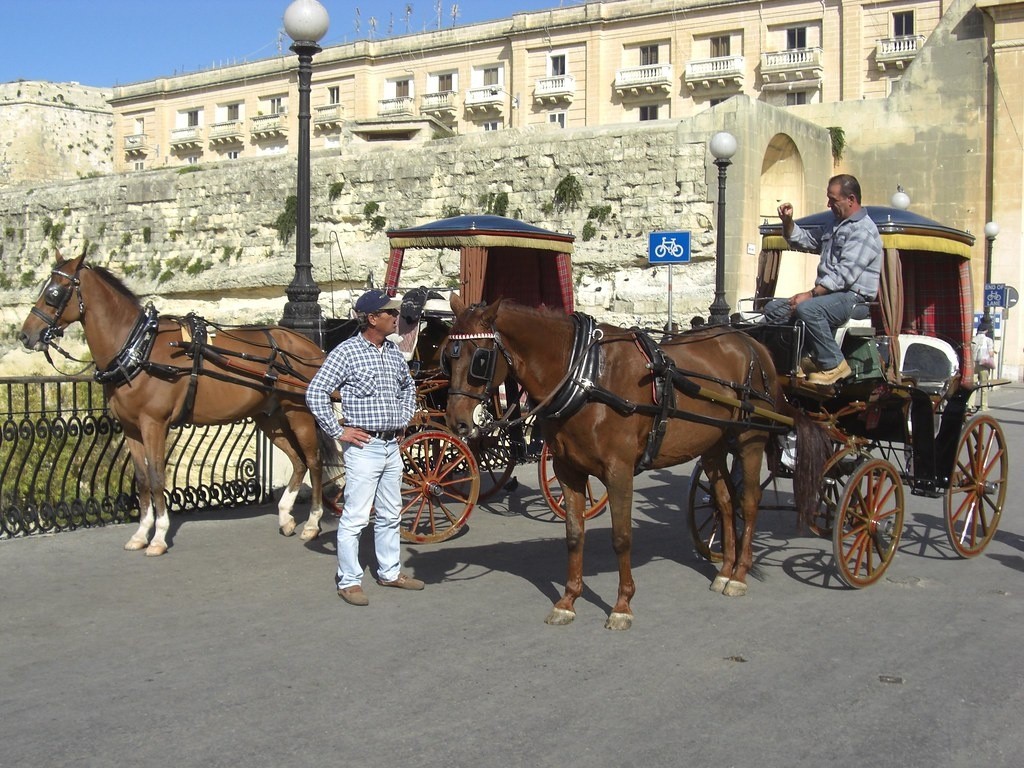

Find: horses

[445,290,841,630]
[19,240,347,557]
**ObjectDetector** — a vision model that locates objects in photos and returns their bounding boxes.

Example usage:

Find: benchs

[737,297,880,353]
[895,334,960,439]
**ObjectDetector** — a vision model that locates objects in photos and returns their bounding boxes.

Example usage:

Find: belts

[363,429,397,441]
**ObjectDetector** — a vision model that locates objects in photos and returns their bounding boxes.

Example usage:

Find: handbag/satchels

[979,350,996,369]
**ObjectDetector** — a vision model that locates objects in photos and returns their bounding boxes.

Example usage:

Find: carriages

[429,202,1011,634]
[14,211,611,558]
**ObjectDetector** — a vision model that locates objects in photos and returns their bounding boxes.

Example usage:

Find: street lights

[707,132,738,327]
[982,221,1000,340]
[274,0,330,346]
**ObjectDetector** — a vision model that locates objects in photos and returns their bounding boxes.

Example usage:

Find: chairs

[390,285,453,362]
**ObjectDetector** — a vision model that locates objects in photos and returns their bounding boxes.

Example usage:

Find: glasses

[378,310,396,315]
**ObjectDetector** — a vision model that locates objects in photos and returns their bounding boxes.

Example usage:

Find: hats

[978,324,987,331]
[354,289,399,314]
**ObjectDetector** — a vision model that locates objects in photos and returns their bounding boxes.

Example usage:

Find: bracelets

[811,289,817,297]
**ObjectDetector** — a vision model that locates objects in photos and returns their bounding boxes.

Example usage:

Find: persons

[980,316,993,339]
[305,290,424,605]
[967,323,997,411]
[764,174,882,385]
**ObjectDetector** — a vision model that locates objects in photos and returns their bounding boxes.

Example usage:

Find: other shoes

[807,358,852,385]
[378,571,428,590]
[336,585,371,607]
[966,409,973,417]
[796,355,820,378]
